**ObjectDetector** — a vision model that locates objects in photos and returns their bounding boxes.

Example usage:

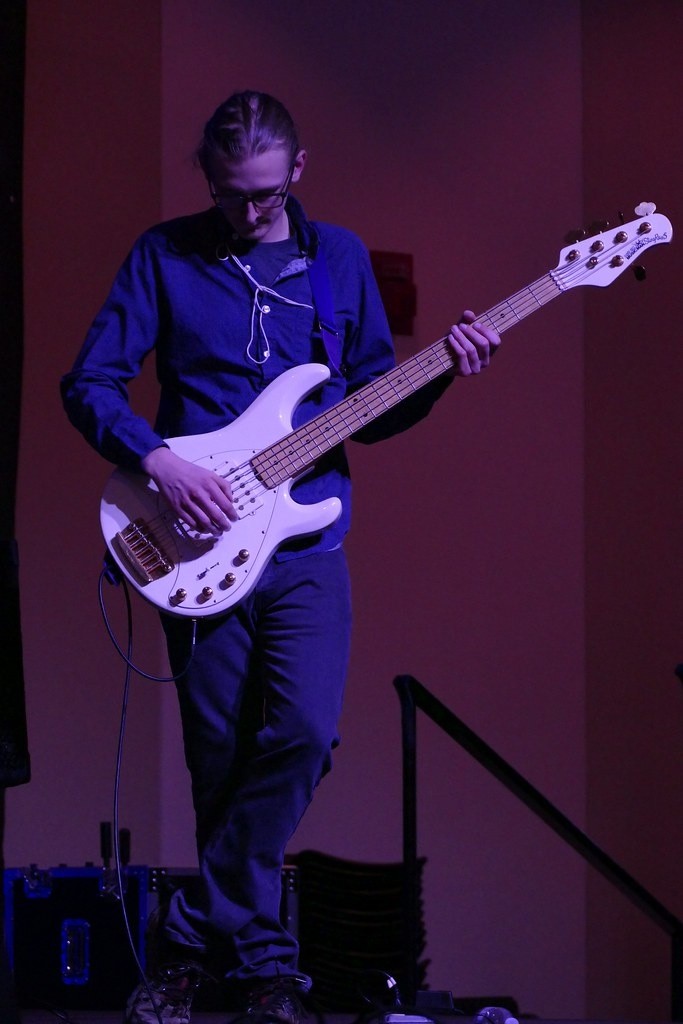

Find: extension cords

[382,1012,433,1024]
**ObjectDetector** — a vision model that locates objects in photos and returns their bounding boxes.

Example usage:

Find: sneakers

[125,901,206,1024]
[231,979,310,1024]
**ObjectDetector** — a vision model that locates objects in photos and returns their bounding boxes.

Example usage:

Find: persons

[57,92,501,1024]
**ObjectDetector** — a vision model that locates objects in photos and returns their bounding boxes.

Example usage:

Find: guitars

[99,199,676,618]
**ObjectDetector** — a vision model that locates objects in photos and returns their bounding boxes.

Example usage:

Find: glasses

[201,159,294,208]
